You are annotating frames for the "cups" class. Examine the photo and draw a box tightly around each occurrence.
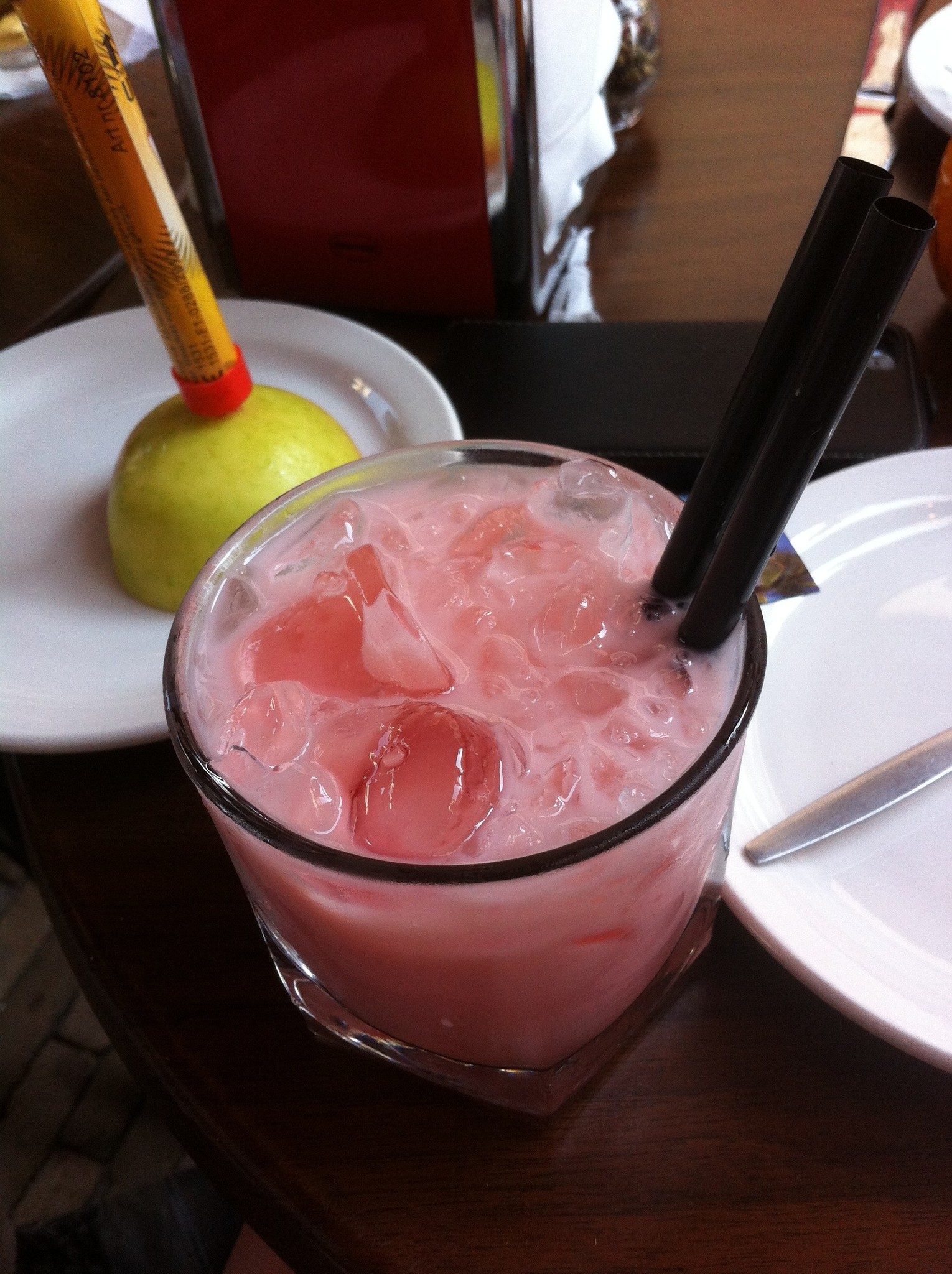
[162,441,769,1117]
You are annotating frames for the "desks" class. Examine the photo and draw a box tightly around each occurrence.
[3,309,951,1273]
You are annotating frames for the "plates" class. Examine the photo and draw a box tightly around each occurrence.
[1,300,466,755]
[722,447,952,1073]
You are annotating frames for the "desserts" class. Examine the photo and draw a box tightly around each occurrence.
[107,381,374,612]
